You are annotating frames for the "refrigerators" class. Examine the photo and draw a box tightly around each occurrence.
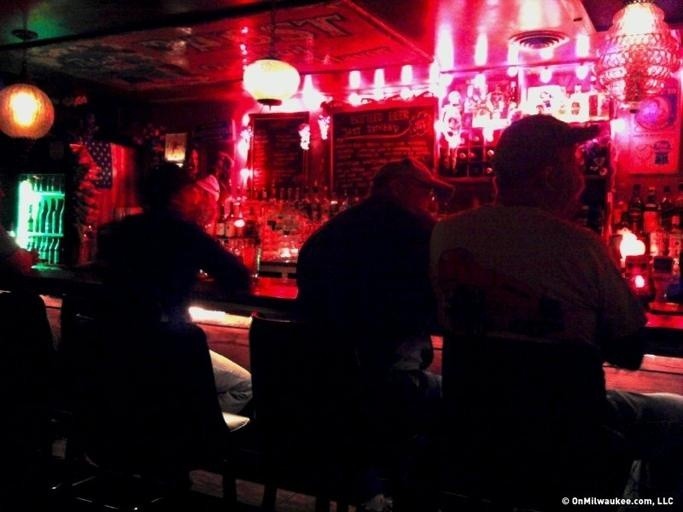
[9,135,136,269]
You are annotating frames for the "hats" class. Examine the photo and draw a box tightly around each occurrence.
[372,153,453,200]
[491,113,600,202]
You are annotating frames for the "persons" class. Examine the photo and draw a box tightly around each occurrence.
[428,111,683,512]
[292,161,455,512]
[91,160,255,493]
[0,181,40,277]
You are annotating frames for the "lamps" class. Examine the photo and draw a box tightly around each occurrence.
[240,0,300,109]
[0,26,54,141]
[596,0,681,115]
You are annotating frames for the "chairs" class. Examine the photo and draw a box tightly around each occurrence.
[216,312,437,510]
[50,277,253,511]
[439,278,649,508]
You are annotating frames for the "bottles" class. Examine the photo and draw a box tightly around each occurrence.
[615,181,683,259]
[23,173,66,264]
[217,182,356,287]
[567,76,600,121]
[441,103,462,147]
